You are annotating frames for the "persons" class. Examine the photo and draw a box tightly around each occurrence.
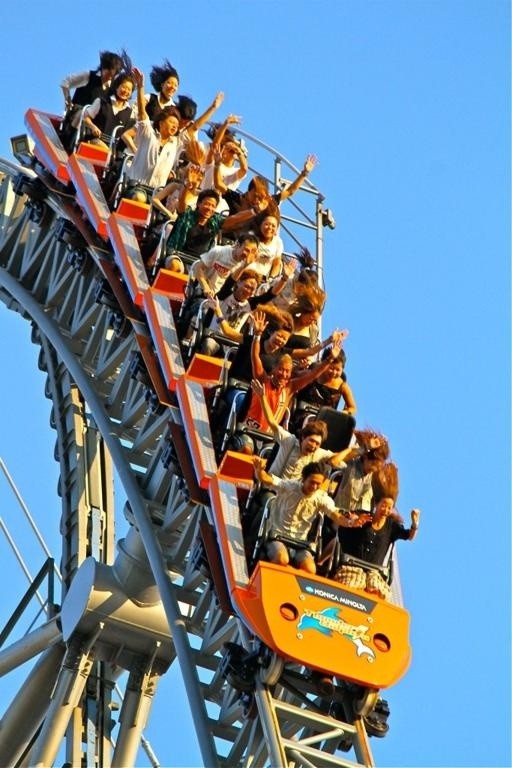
[64,48,421,594]
